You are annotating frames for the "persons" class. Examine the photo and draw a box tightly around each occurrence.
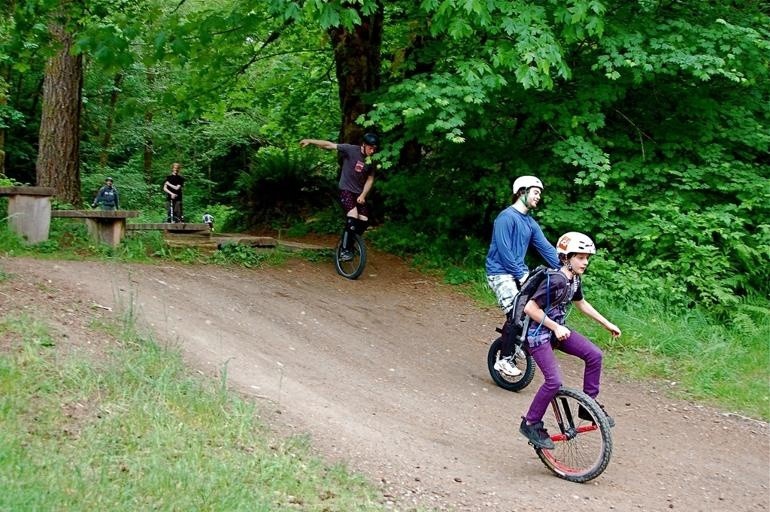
[202,212,216,231]
[162,164,186,224]
[92,177,119,211]
[518,230,622,450]
[484,175,560,376]
[299,132,380,261]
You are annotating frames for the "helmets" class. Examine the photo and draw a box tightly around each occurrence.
[362,132,379,146]
[555,231,598,255]
[511,174,545,196]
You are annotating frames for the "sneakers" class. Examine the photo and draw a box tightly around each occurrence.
[339,246,355,261]
[491,349,522,377]
[513,344,527,359]
[518,415,556,449]
[577,399,617,427]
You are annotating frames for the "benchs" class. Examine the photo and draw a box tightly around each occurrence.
[51,210,139,247]
[0,186,55,244]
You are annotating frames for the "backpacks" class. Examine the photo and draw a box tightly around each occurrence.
[508,263,581,362]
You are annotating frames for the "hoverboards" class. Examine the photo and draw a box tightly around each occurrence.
[527,342,614,483]
[487,326,536,393]
[333,203,368,280]
[163,197,185,223]
